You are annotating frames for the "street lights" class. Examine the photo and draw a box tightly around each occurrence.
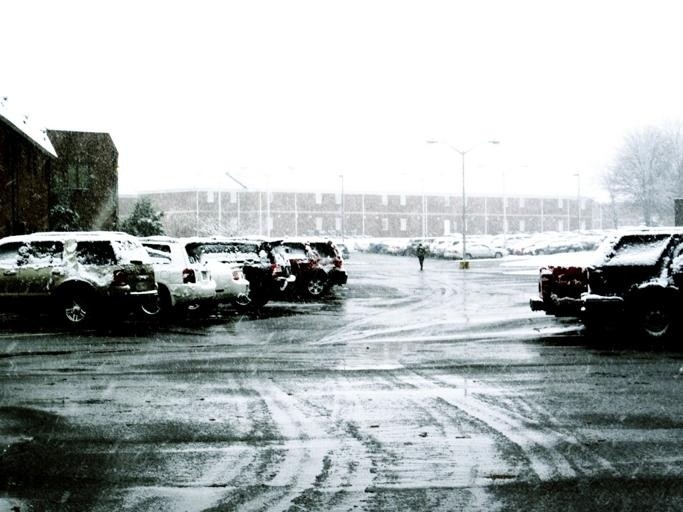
[427,137,500,259]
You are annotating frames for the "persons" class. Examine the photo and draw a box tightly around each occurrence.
[416,244,427,273]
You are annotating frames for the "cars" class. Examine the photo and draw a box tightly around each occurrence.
[355,231,595,259]
[529,225,683,343]
